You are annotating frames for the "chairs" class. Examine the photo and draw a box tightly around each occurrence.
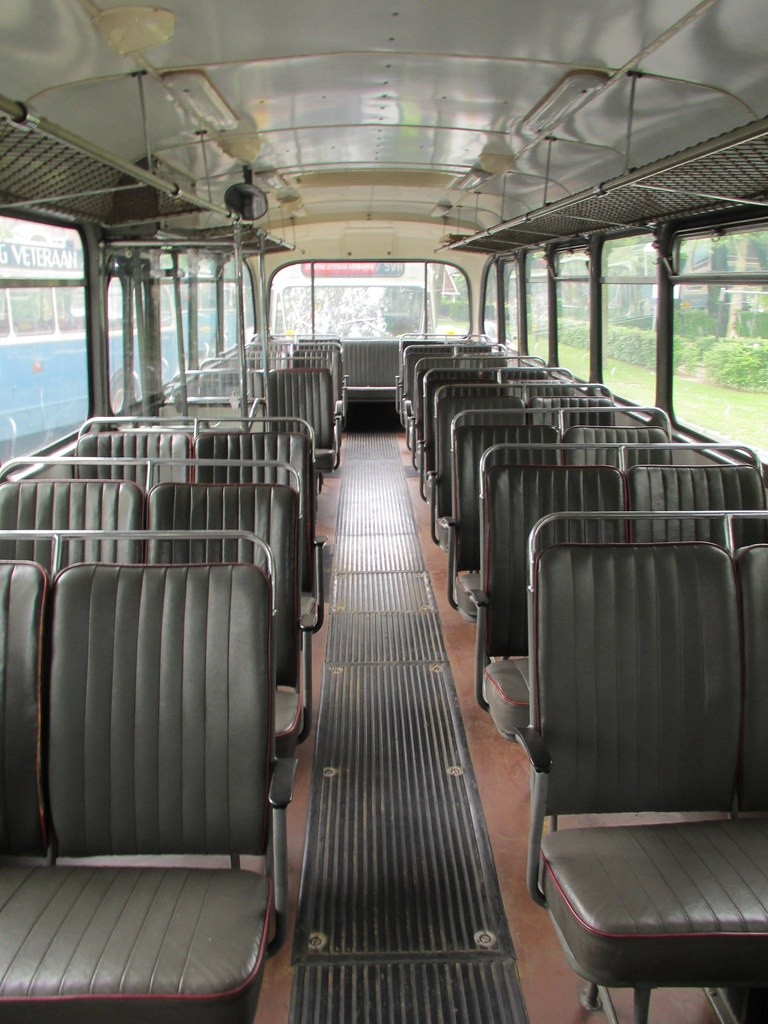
[0,341,768,1024]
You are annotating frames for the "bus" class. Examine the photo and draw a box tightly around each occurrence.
[0,241,245,450]
[267,262,437,354]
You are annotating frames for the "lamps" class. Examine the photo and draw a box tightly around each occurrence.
[522,69,610,136]
[429,204,452,218]
[452,167,491,191]
[161,69,239,132]
[261,173,291,190]
